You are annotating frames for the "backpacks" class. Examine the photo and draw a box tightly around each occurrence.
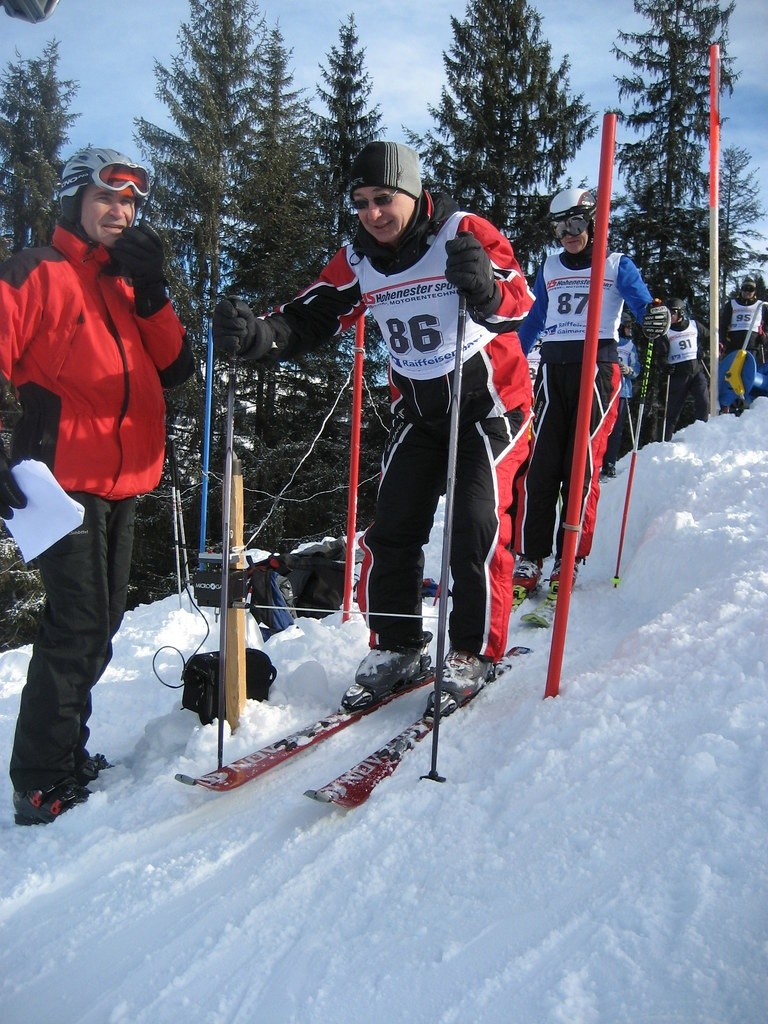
[246,536,346,642]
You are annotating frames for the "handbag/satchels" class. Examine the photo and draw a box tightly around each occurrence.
[181,647,277,726]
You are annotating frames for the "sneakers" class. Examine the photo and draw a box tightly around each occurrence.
[549,556,579,593]
[433,646,490,698]
[73,753,116,783]
[355,648,421,693]
[12,778,93,826]
[601,461,618,478]
[512,555,543,591]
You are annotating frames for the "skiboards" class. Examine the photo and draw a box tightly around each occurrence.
[171,645,533,811]
[509,582,559,627]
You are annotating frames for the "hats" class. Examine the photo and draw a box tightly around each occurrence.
[740,276,756,287]
[349,141,422,198]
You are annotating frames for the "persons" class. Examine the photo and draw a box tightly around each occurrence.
[601,279,768,478]
[0,148,195,826]
[212,141,537,700]
[517,188,671,596]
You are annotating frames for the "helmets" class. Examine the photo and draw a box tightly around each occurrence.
[618,312,631,331]
[60,148,133,206]
[549,189,596,238]
[666,297,686,310]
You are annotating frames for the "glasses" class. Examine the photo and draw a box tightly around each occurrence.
[550,216,590,239]
[625,322,638,330]
[670,309,678,316]
[91,162,150,198]
[350,189,398,210]
[741,285,756,293]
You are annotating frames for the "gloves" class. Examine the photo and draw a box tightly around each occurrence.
[0,440,28,521]
[212,296,258,362]
[755,333,767,345]
[113,219,170,315]
[443,237,501,321]
[618,363,629,375]
[641,303,671,339]
[660,362,676,375]
